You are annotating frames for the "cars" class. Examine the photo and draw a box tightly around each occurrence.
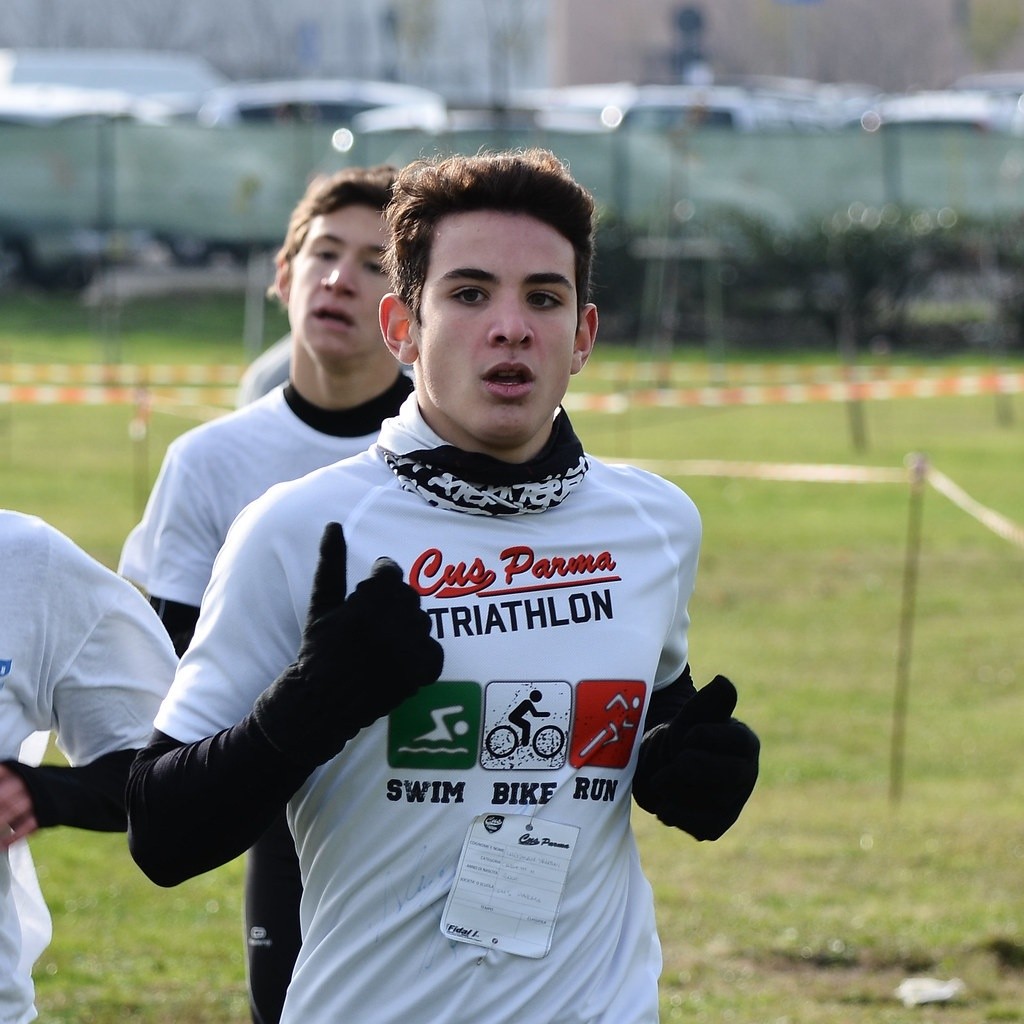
[0,47,1024,302]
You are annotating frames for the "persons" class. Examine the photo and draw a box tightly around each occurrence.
[0,509,183,1024]
[129,148,761,1024]
[117,166,415,1022]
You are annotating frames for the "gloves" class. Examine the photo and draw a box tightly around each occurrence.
[634,674,762,843]
[253,521,445,773]
